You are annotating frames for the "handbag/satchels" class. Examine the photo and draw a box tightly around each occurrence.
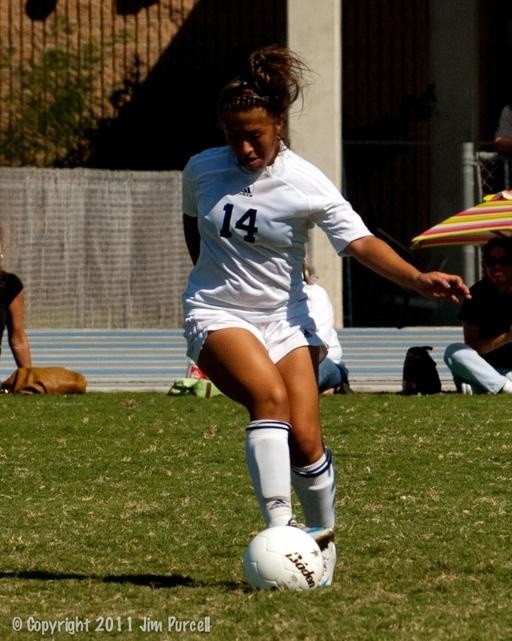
[0,365,89,397]
[165,376,225,399]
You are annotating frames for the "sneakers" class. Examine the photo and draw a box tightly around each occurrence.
[286,517,335,552]
[319,534,337,588]
[333,381,355,394]
[459,382,478,398]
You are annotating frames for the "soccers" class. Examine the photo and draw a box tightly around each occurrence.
[244,525,324,595]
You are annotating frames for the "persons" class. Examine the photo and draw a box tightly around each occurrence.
[176,43,472,593]
[0,245,33,371]
[440,234,511,397]
[301,260,353,397]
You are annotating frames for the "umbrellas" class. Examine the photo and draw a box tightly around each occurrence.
[410,188,512,249]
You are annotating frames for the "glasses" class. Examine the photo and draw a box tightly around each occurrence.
[482,254,511,270]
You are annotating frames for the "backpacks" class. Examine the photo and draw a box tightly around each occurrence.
[397,344,442,396]
